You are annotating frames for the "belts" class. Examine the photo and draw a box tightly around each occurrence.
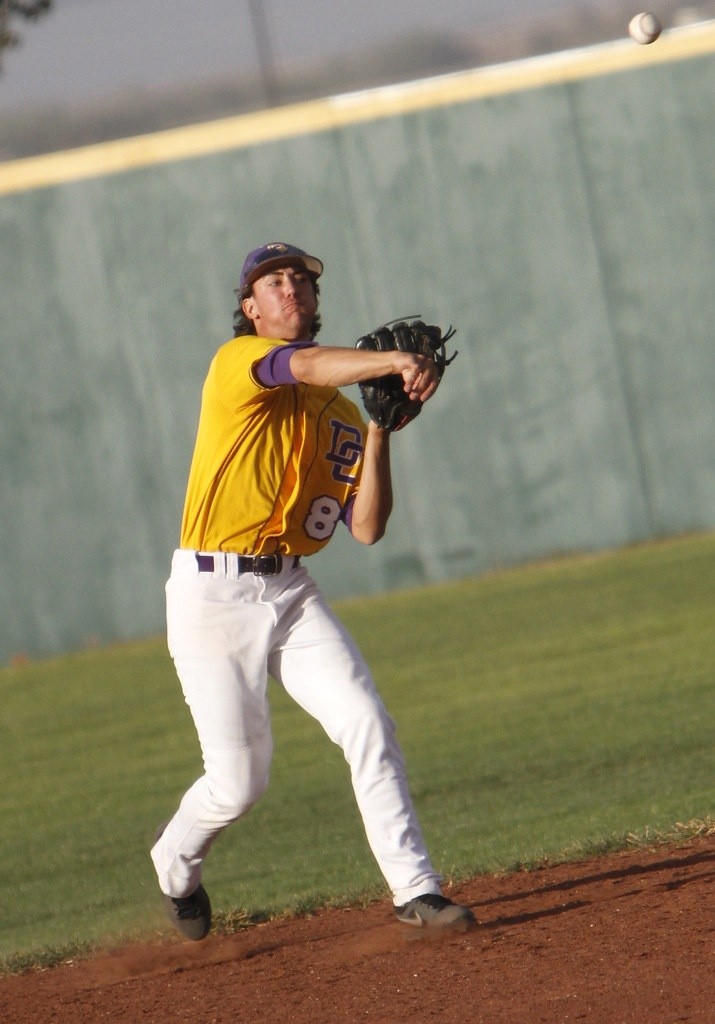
[197,554,301,576]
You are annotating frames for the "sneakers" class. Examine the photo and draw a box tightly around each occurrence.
[153,822,212,940]
[393,893,476,935]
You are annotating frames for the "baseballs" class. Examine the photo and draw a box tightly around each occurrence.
[627,11,663,45]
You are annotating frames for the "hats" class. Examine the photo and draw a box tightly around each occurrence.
[239,241,323,295]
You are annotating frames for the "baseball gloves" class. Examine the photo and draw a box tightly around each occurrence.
[354,319,445,433]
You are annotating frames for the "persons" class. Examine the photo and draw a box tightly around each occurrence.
[149,242,475,943]
